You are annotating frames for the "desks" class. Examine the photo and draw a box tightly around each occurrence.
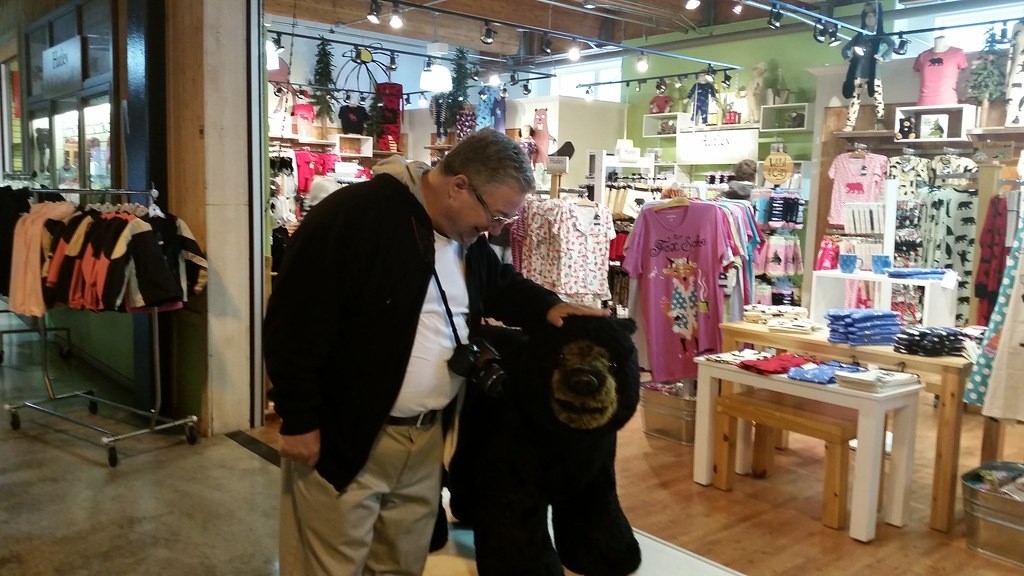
[718,307,1009,534]
[692,348,927,543]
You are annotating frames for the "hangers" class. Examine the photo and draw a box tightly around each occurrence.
[609,176,752,233]
[270,189,298,229]
[0,176,187,231]
[832,145,979,176]
[521,187,609,212]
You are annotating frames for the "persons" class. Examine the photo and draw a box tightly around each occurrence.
[913,36,968,106]
[750,63,769,123]
[842,1,895,132]
[261,133,612,576]
[682,69,720,128]
[715,160,757,200]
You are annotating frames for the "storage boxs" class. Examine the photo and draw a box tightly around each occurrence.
[638,380,696,446]
[962,461,1024,568]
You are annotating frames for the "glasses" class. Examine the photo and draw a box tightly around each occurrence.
[470,182,520,225]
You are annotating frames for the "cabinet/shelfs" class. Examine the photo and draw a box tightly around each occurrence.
[743,188,803,302]
[809,269,959,332]
[753,161,811,200]
[585,143,691,181]
[424,144,455,151]
[840,182,928,328]
[831,104,1024,143]
[269,134,404,158]
[640,102,816,139]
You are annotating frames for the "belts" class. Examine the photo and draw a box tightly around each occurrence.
[385,411,444,428]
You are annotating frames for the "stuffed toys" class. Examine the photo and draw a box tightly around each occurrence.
[426,313,652,576]
[897,116,916,140]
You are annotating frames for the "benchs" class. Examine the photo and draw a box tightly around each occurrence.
[713,389,890,531]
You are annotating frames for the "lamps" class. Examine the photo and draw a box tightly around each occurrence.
[265,1,914,104]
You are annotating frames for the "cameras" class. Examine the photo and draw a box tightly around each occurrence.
[448,337,506,394]
